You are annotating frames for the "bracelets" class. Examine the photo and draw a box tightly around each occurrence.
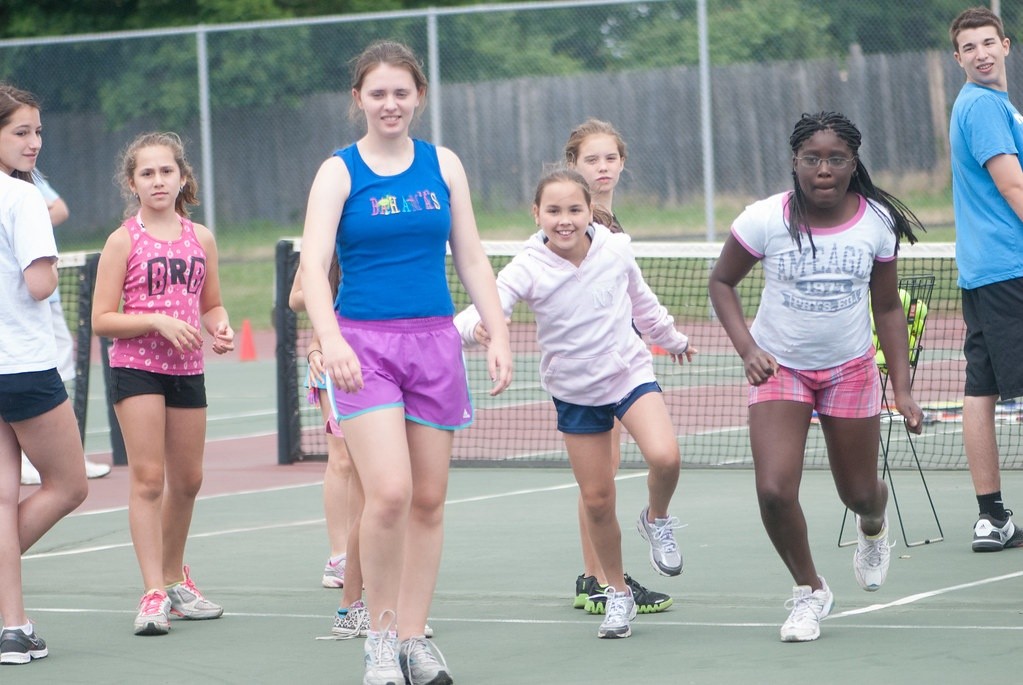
[307,350,322,364]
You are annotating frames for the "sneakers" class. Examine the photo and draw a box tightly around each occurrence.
[322,557,364,590]
[134,589,171,635]
[0,623,48,664]
[972,509,1023,552]
[573,572,673,614]
[165,565,223,619]
[637,506,688,577]
[598,585,637,638]
[333,600,433,640]
[363,609,406,685]
[399,636,453,685]
[781,575,835,642]
[854,511,896,591]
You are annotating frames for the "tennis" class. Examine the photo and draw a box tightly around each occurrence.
[867,286,927,372]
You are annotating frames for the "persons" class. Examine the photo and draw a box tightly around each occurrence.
[287,250,434,638]
[707,112,923,642]
[0,80,109,665]
[451,168,698,641]
[948,9,1022,552]
[563,118,674,613]
[299,39,515,685]
[90,132,234,635]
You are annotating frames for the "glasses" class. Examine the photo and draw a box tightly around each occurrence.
[795,155,855,169]
